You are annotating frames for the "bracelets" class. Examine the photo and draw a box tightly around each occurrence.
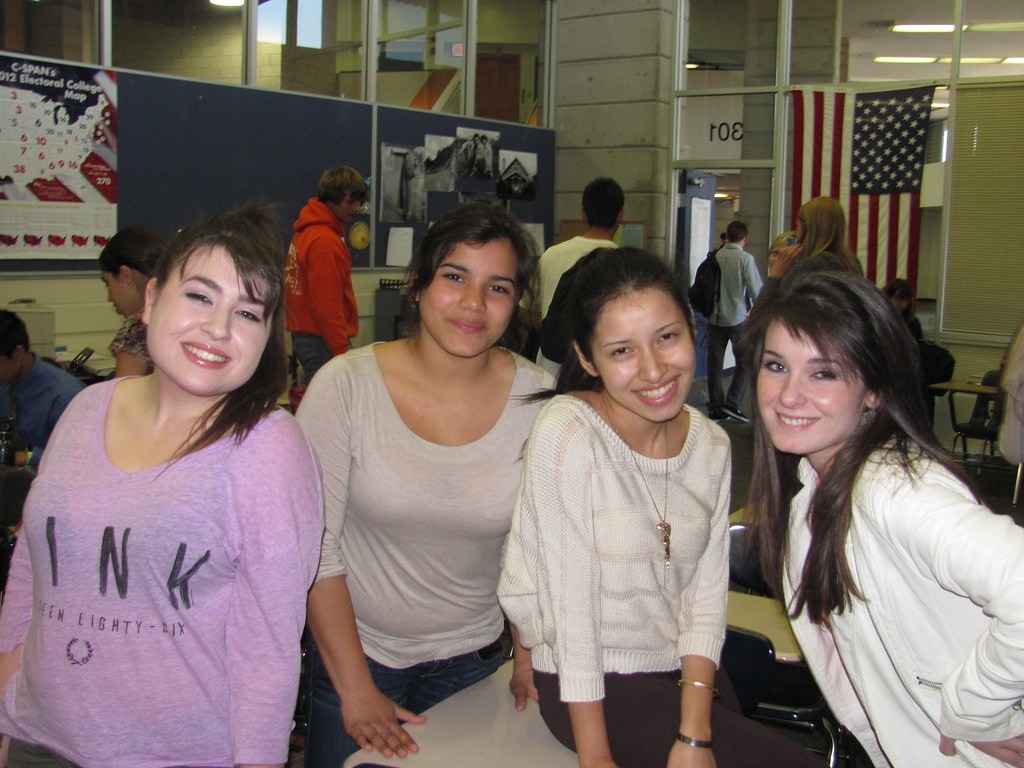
[677,732,712,749]
[678,679,718,697]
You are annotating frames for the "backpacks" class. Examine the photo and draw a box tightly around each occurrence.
[688,249,721,319]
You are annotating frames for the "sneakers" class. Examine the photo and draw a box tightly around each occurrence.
[721,404,750,422]
[708,412,731,423]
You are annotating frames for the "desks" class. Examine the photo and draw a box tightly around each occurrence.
[727,591,803,662]
[344,660,579,768]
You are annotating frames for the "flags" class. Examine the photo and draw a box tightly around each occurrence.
[791,84,934,302]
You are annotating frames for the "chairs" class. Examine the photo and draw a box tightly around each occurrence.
[950,366,1024,507]
[719,522,851,768]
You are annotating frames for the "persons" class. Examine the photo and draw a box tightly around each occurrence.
[719,232,728,245]
[703,220,762,424]
[881,278,934,428]
[996,320,1024,509]
[535,175,626,381]
[748,196,862,329]
[462,133,496,178]
[496,245,829,768]
[95,224,168,379]
[296,200,559,768]
[283,165,369,383]
[0,309,87,495]
[0,202,326,768]
[730,271,1023,767]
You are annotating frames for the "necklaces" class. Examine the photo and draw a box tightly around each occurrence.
[600,391,672,565]
